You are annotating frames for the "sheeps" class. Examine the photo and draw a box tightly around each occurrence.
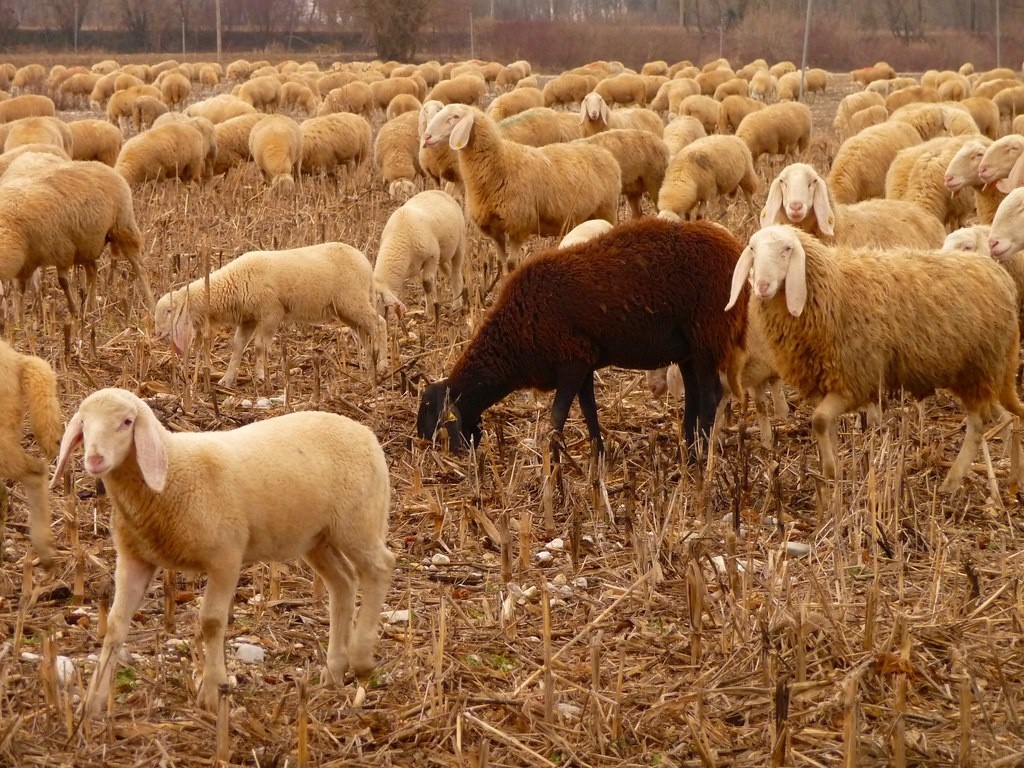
[1,53,1024,502]
[44,384,397,716]
